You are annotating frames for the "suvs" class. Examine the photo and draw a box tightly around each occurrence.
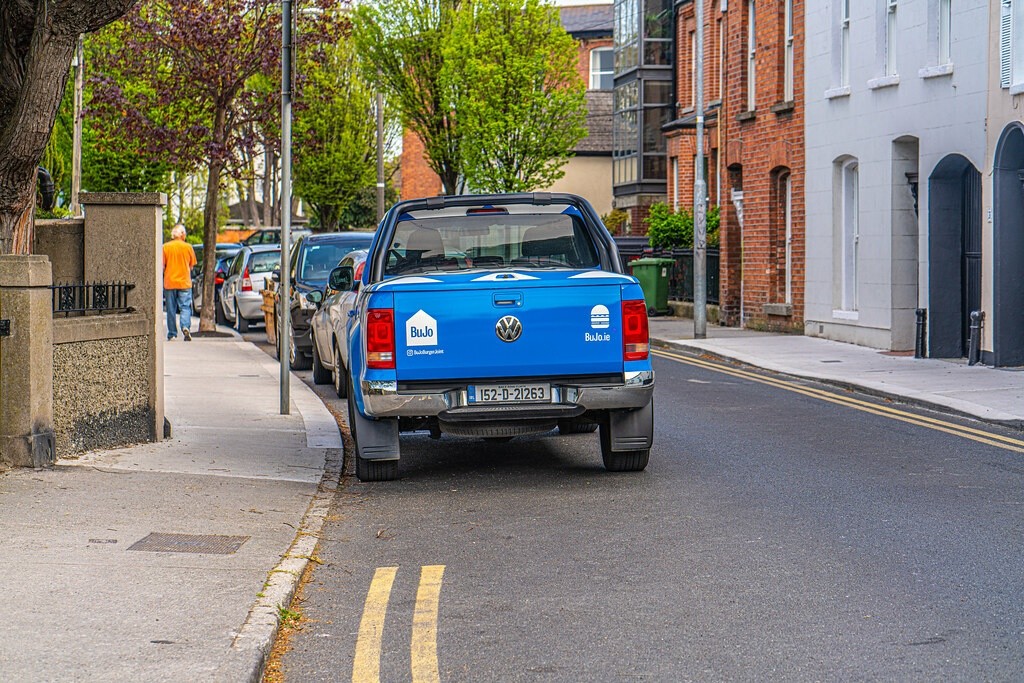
[270,231,401,371]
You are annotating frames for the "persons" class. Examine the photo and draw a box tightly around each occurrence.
[163,225,197,342]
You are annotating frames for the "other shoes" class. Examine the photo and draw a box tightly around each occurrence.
[169,336,176,340]
[182,326,191,341]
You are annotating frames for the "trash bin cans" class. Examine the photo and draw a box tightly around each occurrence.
[627,257,676,317]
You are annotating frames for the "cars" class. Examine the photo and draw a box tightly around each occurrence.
[305,246,474,399]
[161,227,312,320]
[217,242,297,333]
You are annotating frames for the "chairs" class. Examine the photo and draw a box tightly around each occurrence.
[522,225,572,267]
[398,228,458,274]
[308,249,331,278]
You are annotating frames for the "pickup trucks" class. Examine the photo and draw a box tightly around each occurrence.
[326,191,658,484]
